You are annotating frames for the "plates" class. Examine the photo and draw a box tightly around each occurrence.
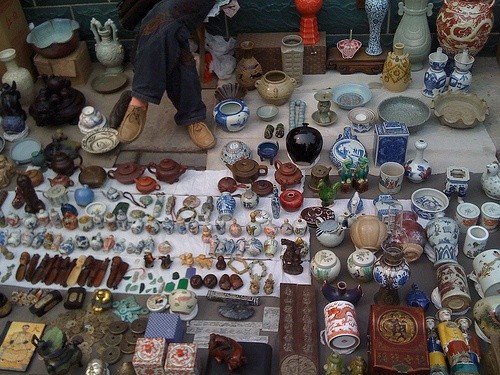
[82,128,121,154]
[10,138,41,163]
[91,72,128,94]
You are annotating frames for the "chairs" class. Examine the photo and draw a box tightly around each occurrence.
[195,0,229,89]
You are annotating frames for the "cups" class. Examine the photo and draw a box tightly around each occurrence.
[463,226,489,258]
[473,249,500,297]
[379,162,405,194]
[437,264,471,313]
[455,203,480,233]
[324,301,360,353]
[480,202,500,232]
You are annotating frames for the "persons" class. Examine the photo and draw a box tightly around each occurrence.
[117,0,217,150]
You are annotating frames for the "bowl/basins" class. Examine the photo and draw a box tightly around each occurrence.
[331,84,372,110]
[377,96,431,130]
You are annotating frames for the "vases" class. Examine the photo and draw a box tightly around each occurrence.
[349,215,389,251]
[373,242,412,305]
[397,211,426,262]
[0,48,36,115]
[426,212,459,268]
[216,192,235,221]
[236,41,262,91]
[212,98,249,132]
[364,0,496,93]
[294,0,324,45]
[404,139,431,183]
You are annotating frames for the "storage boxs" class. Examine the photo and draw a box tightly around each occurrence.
[143,312,185,343]
[0,0,29,72]
[132,337,167,375]
[164,343,201,375]
[234,31,326,74]
[33,40,91,85]
[366,304,431,375]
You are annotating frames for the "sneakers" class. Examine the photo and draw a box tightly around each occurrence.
[185,122,215,149]
[117,103,148,144]
